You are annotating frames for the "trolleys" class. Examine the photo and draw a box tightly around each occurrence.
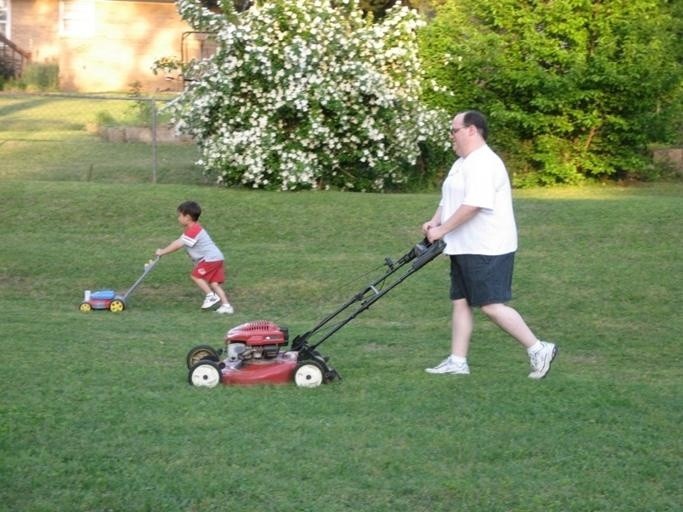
[76,250,165,313]
[185,230,447,395]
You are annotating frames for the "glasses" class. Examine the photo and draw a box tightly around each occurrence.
[450,125,469,137]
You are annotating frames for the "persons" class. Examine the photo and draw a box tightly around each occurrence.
[154,202,236,314]
[421,110,556,380]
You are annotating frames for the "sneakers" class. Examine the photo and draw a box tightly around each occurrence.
[527,341,558,379]
[425,356,470,375]
[214,304,234,315]
[200,291,221,309]
